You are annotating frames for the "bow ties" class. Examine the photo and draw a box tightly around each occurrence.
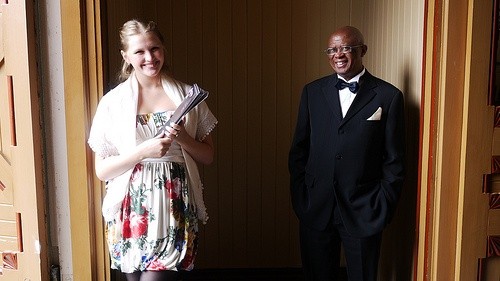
[336,78,357,94]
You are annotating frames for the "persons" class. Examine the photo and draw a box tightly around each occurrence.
[287,27,405,281]
[88,18,222,280]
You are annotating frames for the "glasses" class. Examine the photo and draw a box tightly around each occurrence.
[323,44,363,55]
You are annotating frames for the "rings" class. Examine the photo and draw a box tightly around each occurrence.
[175,132,179,137]
